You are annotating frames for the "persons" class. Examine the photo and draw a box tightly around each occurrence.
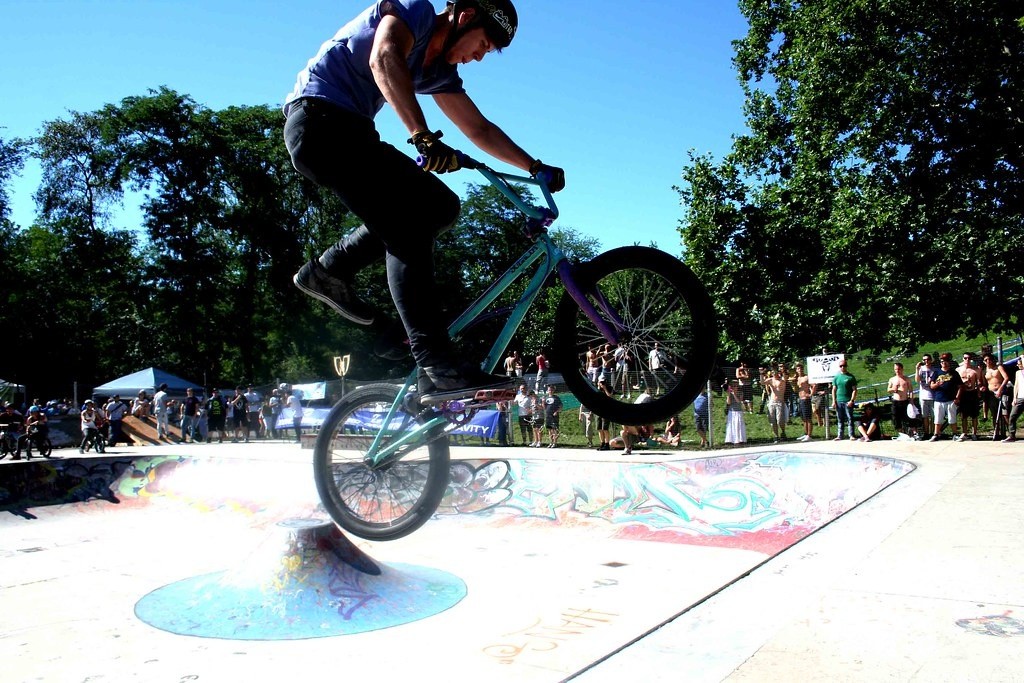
[0,398,81,460]
[736,361,753,414]
[479,405,491,446]
[831,360,857,441]
[282,0,565,405]
[79,383,304,454]
[725,380,747,444]
[887,343,1024,443]
[586,343,678,400]
[693,387,714,447]
[579,381,681,455]
[504,351,522,377]
[495,401,508,447]
[857,402,882,442]
[535,351,548,395]
[452,434,465,445]
[759,361,832,444]
[514,384,563,448]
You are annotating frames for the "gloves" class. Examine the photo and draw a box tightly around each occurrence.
[413,131,463,174]
[529,159,565,193]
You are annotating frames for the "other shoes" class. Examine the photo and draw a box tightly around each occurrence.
[295,440,301,444]
[621,447,632,455]
[11,454,21,460]
[159,434,251,444]
[519,435,1015,448]
[79,447,83,454]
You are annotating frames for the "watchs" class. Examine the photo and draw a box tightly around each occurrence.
[850,400,854,402]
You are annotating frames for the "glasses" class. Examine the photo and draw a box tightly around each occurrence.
[983,358,991,362]
[923,359,930,362]
[840,365,846,368]
[963,357,972,360]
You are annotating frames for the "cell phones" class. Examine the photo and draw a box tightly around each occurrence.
[920,363,926,365]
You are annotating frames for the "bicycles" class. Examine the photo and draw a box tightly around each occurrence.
[82,419,108,453]
[313,150,719,541]
[0,421,53,461]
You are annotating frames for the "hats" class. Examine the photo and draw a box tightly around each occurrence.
[212,387,218,393]
[247,384,253,387]
[940,354,949,360]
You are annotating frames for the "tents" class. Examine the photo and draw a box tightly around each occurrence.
[93,367,203,400]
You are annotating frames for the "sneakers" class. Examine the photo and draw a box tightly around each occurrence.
[293,257,376,325]
[418,361,515,405]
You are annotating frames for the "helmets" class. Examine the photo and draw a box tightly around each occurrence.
[447,0,518,48]
[30,406,39,412]
[85,399,92,405]
